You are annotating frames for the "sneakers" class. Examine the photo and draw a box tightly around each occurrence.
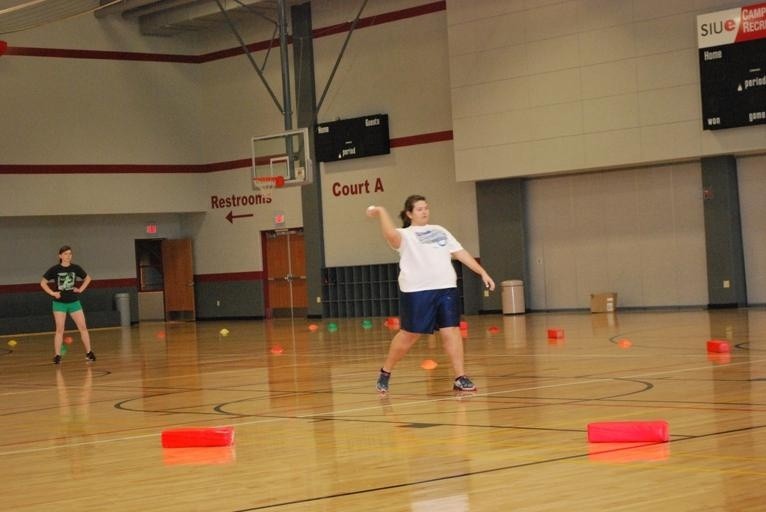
[377,368,389,392]
[86,352,96,361]
[453,375,476,390]
[53,355,61,363]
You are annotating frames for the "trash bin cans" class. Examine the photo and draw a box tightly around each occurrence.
[115,293,132,326]
[501,279,525,314]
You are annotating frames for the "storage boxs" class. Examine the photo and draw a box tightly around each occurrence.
[589,290,618,314]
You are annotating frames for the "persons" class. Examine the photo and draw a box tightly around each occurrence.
[46,361,100,478]
[366,193,496,395]
[377,389,480,511]
[40,244,97,364]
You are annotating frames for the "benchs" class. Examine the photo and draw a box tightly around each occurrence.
[1,288,121,335]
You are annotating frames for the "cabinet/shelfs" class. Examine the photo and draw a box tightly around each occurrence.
[320,260,482,317]
